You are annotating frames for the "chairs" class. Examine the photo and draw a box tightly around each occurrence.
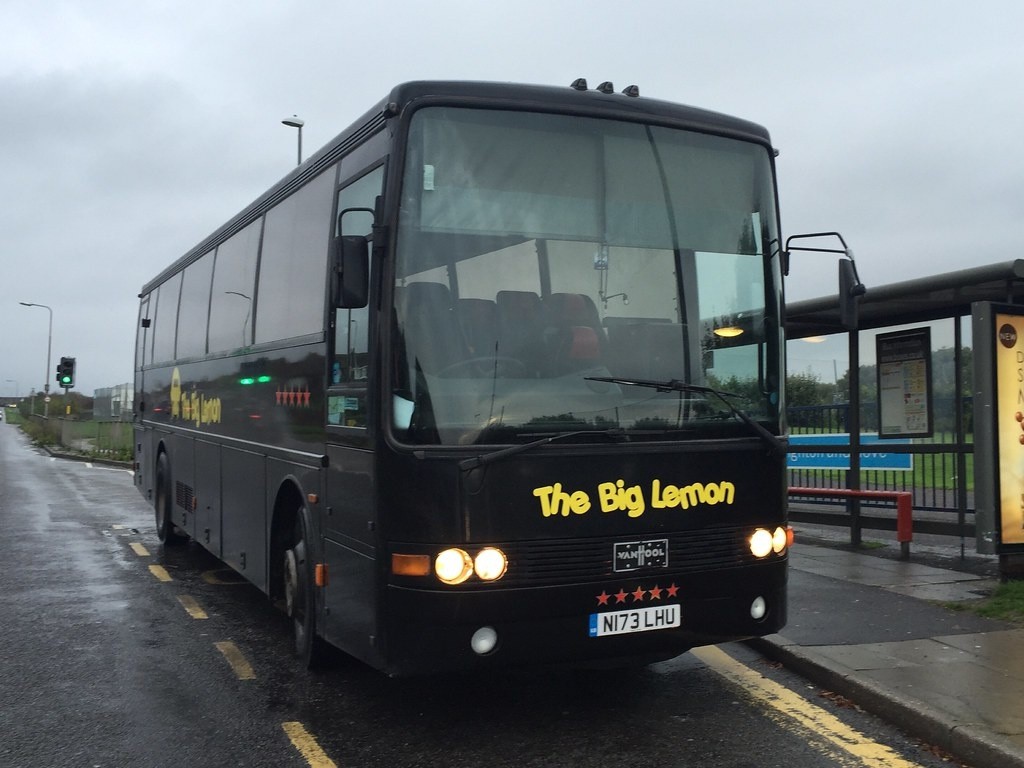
[396,282,609,382]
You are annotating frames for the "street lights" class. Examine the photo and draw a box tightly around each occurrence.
[279,114,304,168]
[18,302,53,417]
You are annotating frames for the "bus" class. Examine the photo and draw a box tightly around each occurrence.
[132,79,866,681]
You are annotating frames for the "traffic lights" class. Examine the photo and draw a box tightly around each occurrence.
[56,358,60,385]
[61,358,75,387]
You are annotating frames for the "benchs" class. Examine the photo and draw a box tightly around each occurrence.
[789,487,913,558]
